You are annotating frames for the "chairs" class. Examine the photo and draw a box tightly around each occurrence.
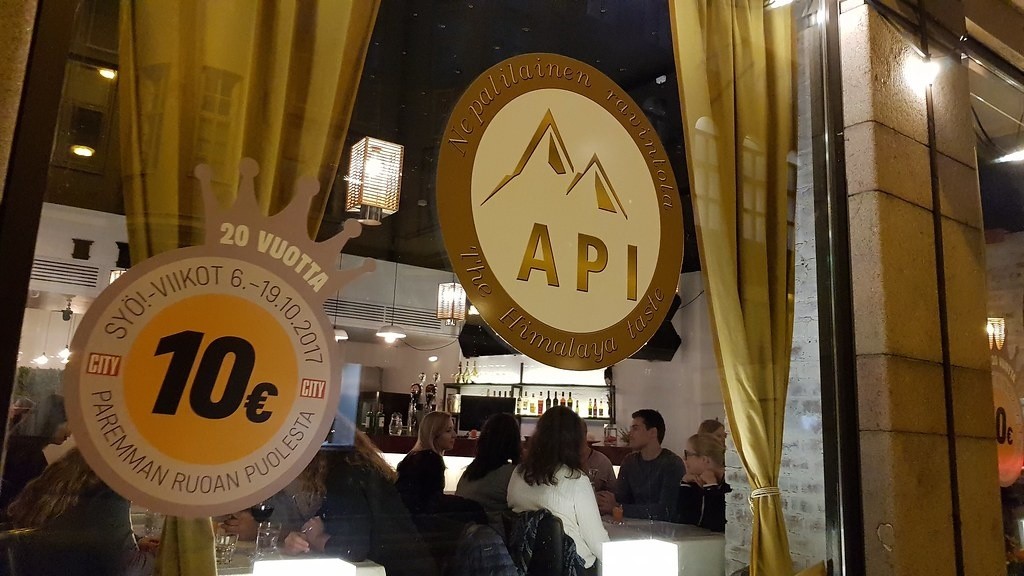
[0,522,119,576]
[411,489,588,575]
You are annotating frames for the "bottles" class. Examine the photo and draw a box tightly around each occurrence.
[599,401,603,417]
[517,391,536,414]
[560,392,579,415]
[389,414,402,436]
[328,430,335,443]
[588,399,597,418]
[486,389,512,398]
[454,361,479,383]
[538,390,558,416]
[366,404,385,436]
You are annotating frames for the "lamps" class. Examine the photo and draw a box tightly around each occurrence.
[36,310,77,365]
[375,239,406,344]
[347,81,404,226]
[109,268,127,285]
[437,271,466,326]
[332,251,348,343]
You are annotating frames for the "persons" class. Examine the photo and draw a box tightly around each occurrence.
[595,409,732,534]
[454,412,524,504]
[0,393,437,576]
[507,406,617,576]
[396,411,457,496]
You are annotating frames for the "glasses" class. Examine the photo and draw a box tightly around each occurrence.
[685,450,710,460]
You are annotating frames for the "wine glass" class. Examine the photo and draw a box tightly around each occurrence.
[247,496,275,557]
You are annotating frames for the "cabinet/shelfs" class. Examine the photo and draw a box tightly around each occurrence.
[443,383,616,424]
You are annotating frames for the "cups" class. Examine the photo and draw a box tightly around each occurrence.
[215,534,239,565]
[257,522,282,555]
[613,504,623,521]
[144,510,166,538]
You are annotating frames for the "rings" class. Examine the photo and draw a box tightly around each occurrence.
[302,528,307,533]
[309,526,312,531]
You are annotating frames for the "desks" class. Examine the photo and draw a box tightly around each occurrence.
[602,514,727,576]
[133,522,385,576]
